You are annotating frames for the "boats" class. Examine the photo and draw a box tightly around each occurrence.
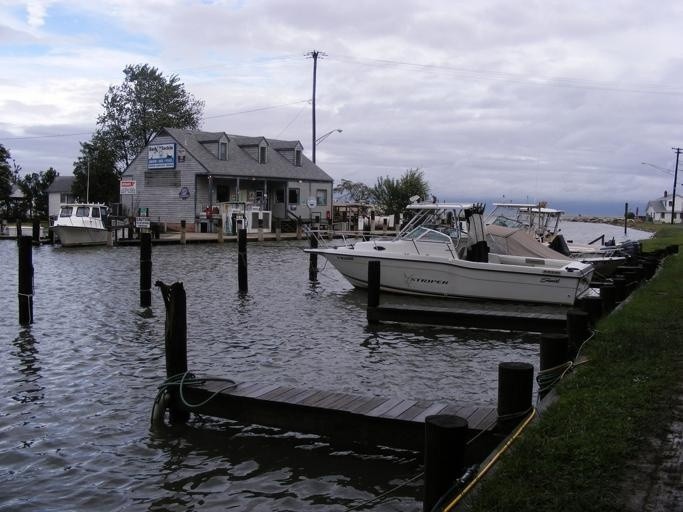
[49,201,109,246]
[303,201,602,305]
[483,203,631,289]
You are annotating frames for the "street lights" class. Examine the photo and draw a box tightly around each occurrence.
[642,161,680,223]
[312,128,343,166]
